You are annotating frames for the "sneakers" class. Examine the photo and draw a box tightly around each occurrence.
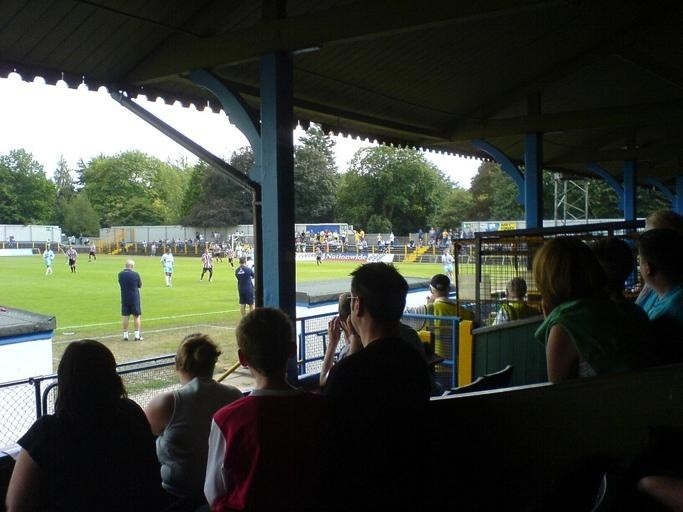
[122,331,129,341]
[134,331,144,341]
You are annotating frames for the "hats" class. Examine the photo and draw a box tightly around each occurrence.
[430,273,451,291]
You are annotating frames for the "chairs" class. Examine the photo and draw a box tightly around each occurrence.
[305,243,477,254]
[1,242,59,253]
[104,242,207,255]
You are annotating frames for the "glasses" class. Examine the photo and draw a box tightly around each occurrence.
[346,295,357,305]
